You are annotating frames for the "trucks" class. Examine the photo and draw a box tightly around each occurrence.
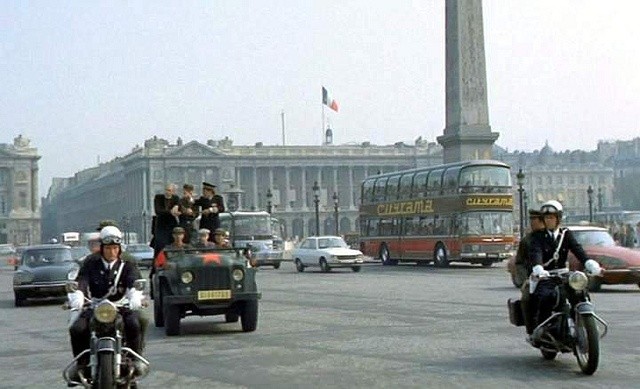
[60,232,81,247]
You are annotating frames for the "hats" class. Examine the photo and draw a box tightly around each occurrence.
[528,209,542,219]
[172,227,185,233]
[203,182,216,190]
[95,219,120,231]
[212,228,226,235]
[198,228,210,234]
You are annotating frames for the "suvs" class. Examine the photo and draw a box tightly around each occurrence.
[150,210,260,335]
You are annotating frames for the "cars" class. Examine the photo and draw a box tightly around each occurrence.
[292,236,363,273]
[562,225,640,292]
[12,244,80,308]
[125,244,158,267]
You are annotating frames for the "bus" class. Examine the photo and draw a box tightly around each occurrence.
[265,218,284,269]
[218,212,271,268]
[358,159,515,268]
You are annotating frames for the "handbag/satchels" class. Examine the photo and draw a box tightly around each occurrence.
[508,298,525,326]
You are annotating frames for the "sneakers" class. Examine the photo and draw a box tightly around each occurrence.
[68,366,91,387]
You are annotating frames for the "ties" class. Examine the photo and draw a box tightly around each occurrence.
[551,231,555,240]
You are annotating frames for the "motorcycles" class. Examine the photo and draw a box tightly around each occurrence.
[507,262,607,377]
[64,278,149,389]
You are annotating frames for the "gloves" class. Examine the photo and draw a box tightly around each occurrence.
[584,259,605,277]
[532,264,550,279]
[67,290,85,309]
[129,291,144,311]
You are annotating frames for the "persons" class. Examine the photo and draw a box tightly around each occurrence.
[362,178,509,236]
[212,227,233,249]
[516,208,546,280]
[66,219,149,389]
[527,200,603,343]
[155,228,192,268]
[154,183,180,251]
[191,229,215,249]
[609,220,640,247]
[195,182,225,233]
[178,184,195,241]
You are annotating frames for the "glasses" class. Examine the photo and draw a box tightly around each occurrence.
[541,205,557,213]
[101,236,122,245]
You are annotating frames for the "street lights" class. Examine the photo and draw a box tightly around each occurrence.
[311,181,322,236]
[265,188,274,214]
[517,169,525,239]
[331,192,340,235]
[587,186,594,223]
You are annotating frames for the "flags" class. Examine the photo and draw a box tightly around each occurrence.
[322,87,338,112]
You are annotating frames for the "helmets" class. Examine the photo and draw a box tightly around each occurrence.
[540,200,563,219]
[99,226,123,253]
